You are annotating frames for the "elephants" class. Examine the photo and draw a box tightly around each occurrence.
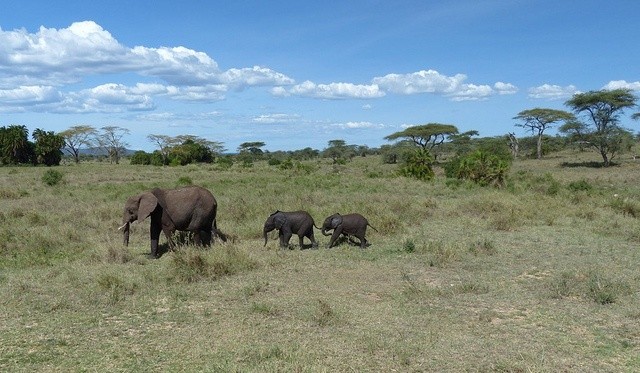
[322,212,379,250]
[117,185,227,261]
[262,209,322,252]
